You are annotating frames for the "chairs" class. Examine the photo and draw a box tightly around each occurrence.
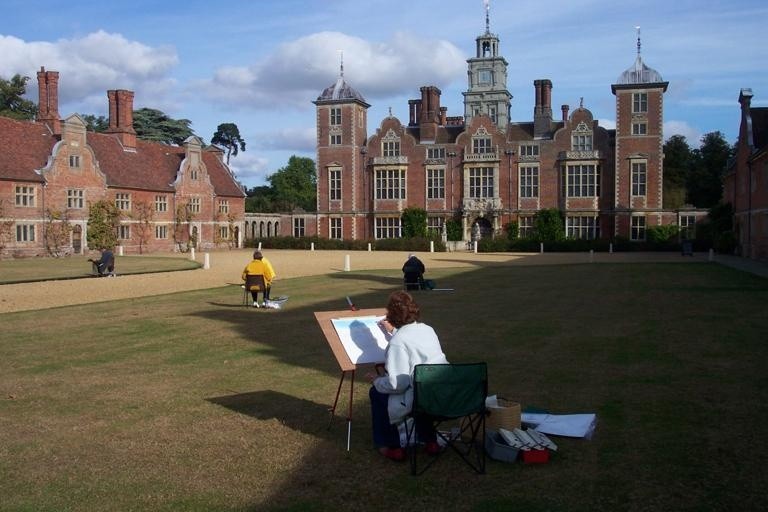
[403,264,425,293]
[404,362,490,474]
[242,275,267,309]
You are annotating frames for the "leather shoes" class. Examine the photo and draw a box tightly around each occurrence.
[379,446,405,461]
[262,302,269,307]
[254,302,259,308]
[424,442,440,456]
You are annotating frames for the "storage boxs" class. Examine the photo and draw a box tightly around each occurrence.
[485,431,519,462]
[524,449,549,464]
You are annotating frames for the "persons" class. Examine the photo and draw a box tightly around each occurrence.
[370,290,450,461]
[242,252,275,309]
[403,253,425,289]
